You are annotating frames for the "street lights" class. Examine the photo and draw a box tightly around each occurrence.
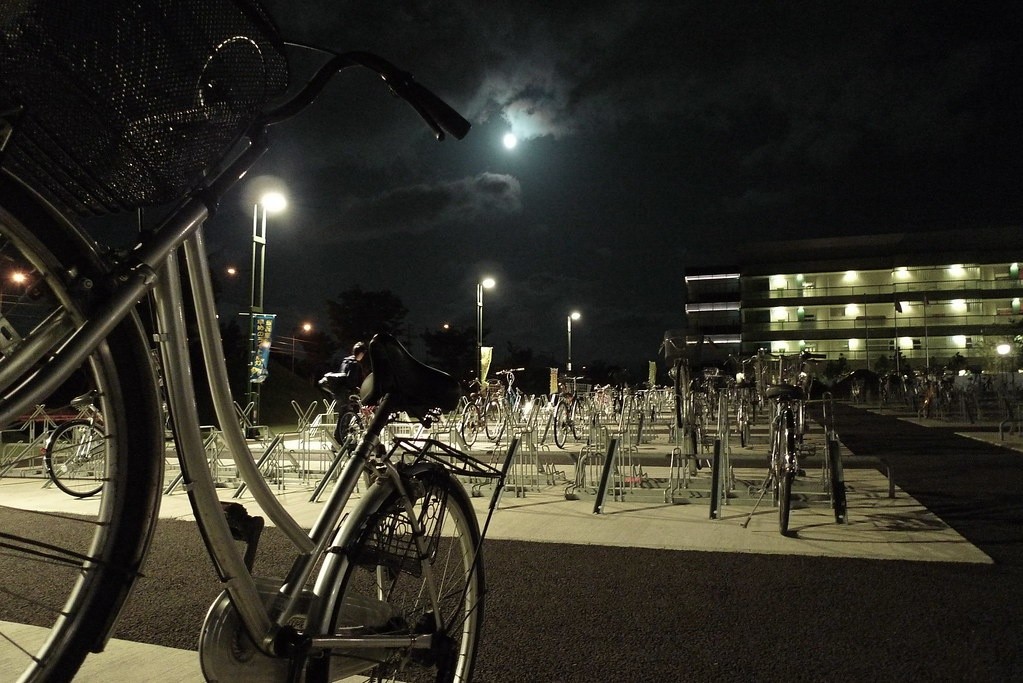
[246,191,287,425]
[475,277,496,379]
[567,312,581,377]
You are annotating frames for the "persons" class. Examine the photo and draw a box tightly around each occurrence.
[328,341,367,451]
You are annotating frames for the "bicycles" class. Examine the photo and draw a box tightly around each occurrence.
[45,347,183,497]
[0,26,514,683]
[302,346,1023,536]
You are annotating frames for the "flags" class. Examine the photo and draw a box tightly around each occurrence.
[896,296,902,313]
